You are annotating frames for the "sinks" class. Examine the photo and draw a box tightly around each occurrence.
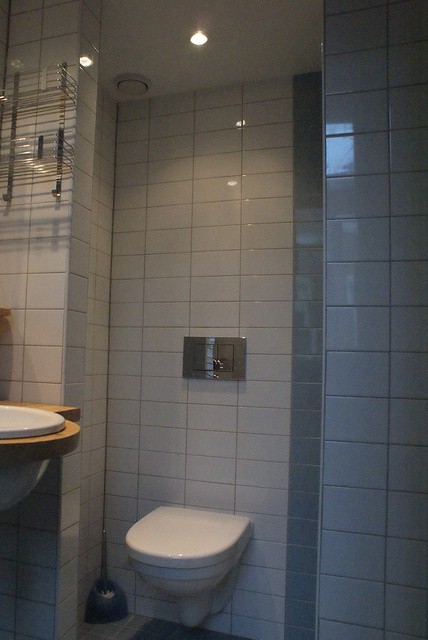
[0,400,81,512]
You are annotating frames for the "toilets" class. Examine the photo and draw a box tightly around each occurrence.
[124,505,254,628]
